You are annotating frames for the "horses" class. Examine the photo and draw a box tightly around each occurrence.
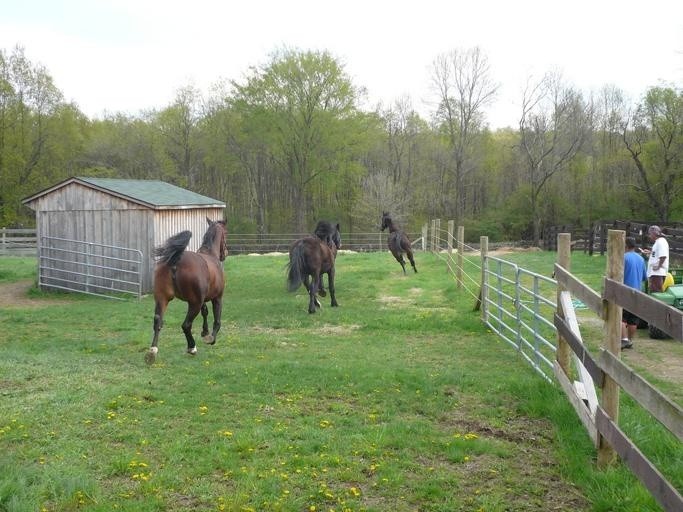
[281,220,343,312]
[144,213,229,367]
[380,210,419,276]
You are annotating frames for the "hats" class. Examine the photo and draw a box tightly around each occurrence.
[649,226,666,237]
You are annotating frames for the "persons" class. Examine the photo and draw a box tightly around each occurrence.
[647,225,669,295]
[621,238,647,348]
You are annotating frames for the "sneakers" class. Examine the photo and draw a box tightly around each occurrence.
[621,339,632,349]
